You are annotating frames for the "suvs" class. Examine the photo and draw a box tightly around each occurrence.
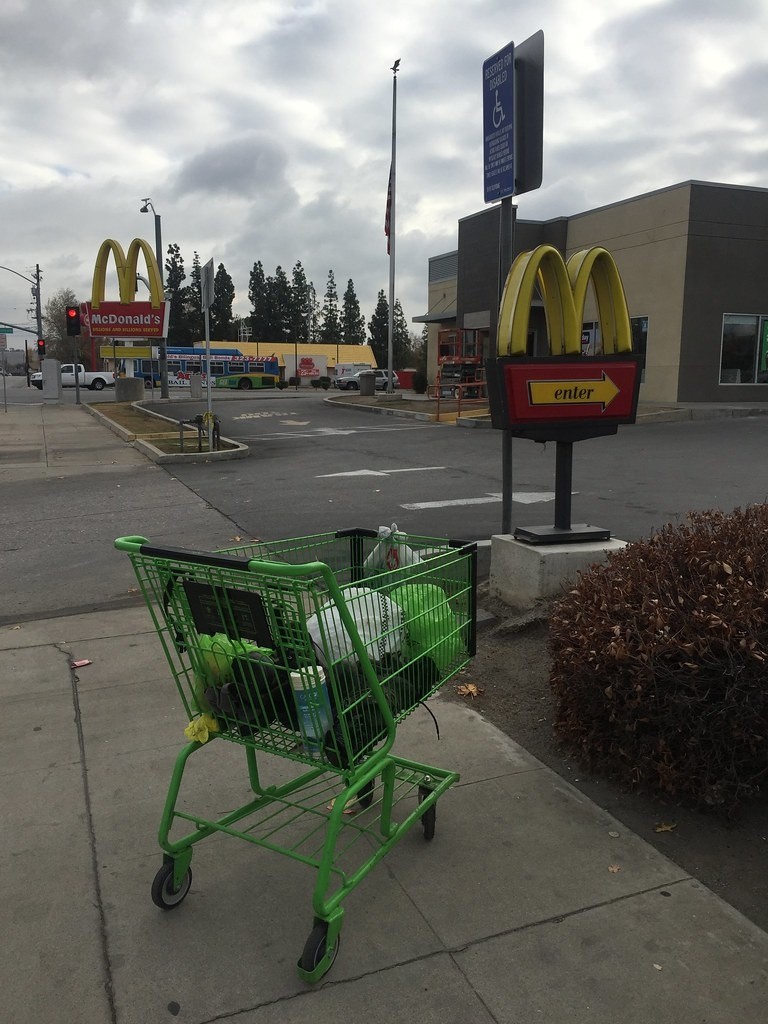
[336,368,400,391]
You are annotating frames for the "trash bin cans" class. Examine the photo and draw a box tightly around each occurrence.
[190,375,202,398]
[359,374,377,396]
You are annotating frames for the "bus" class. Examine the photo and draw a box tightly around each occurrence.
[112,346,281,392]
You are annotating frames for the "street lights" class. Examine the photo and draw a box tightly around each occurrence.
[139,196,163,286]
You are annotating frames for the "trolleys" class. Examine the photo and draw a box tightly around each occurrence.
[114,524,479,986]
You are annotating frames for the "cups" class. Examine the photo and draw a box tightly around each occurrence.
[292,666,333,759]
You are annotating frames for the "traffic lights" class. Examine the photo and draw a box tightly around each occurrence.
[65,305,81,336]
[37,339,45,355]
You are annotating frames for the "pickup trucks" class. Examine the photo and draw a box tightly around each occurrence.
[30,364,116,390]
